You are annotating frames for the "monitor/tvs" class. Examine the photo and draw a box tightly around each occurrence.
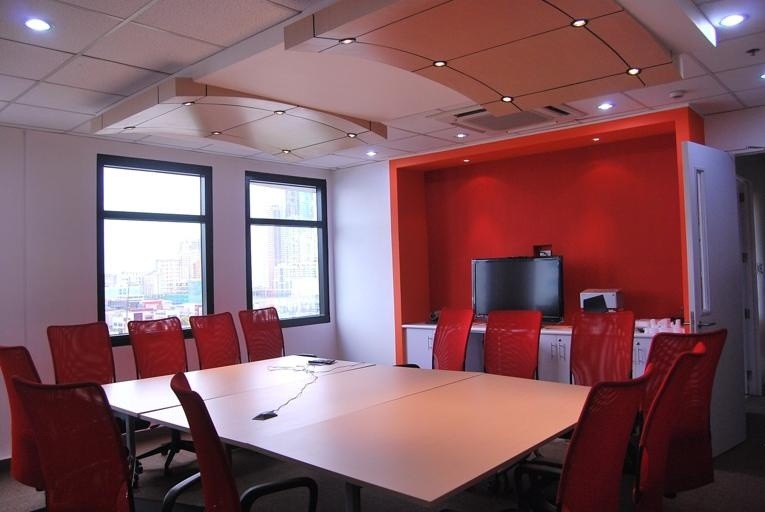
[471,256,564,325]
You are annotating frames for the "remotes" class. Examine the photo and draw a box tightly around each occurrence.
[308,359,335,365]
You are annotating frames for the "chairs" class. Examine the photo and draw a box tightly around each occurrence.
[635,339,709,512]
[238,307,285,362]
[432,308,476,370]
[127,316,196,479]
[645,330,727,398]
[169,371,319,512]
[483,309,544,380]
[46,321,143,490]
[9,375,205,511]
[0,345,46,494]
[569,311,637,383]
[558,362,659,510]
[189,312,241,370]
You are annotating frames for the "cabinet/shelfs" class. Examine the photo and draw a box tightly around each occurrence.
[402,321,485,372]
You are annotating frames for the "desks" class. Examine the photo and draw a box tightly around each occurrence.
[102,352,484,450]
[250,373,601,512]
